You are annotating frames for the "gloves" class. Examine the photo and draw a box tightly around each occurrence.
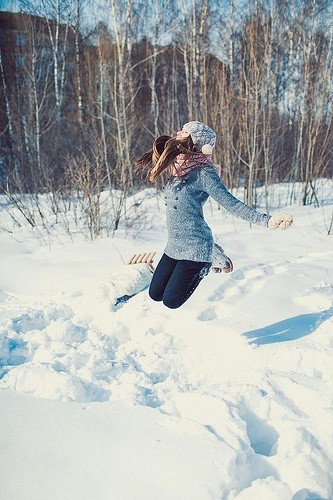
[268,213,293,230]
[212,164,221,177]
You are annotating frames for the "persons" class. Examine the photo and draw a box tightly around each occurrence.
[136,120,294,308]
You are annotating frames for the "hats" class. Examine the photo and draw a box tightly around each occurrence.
[183,121,217,153]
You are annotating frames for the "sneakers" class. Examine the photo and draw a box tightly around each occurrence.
[211,243,233,273]
